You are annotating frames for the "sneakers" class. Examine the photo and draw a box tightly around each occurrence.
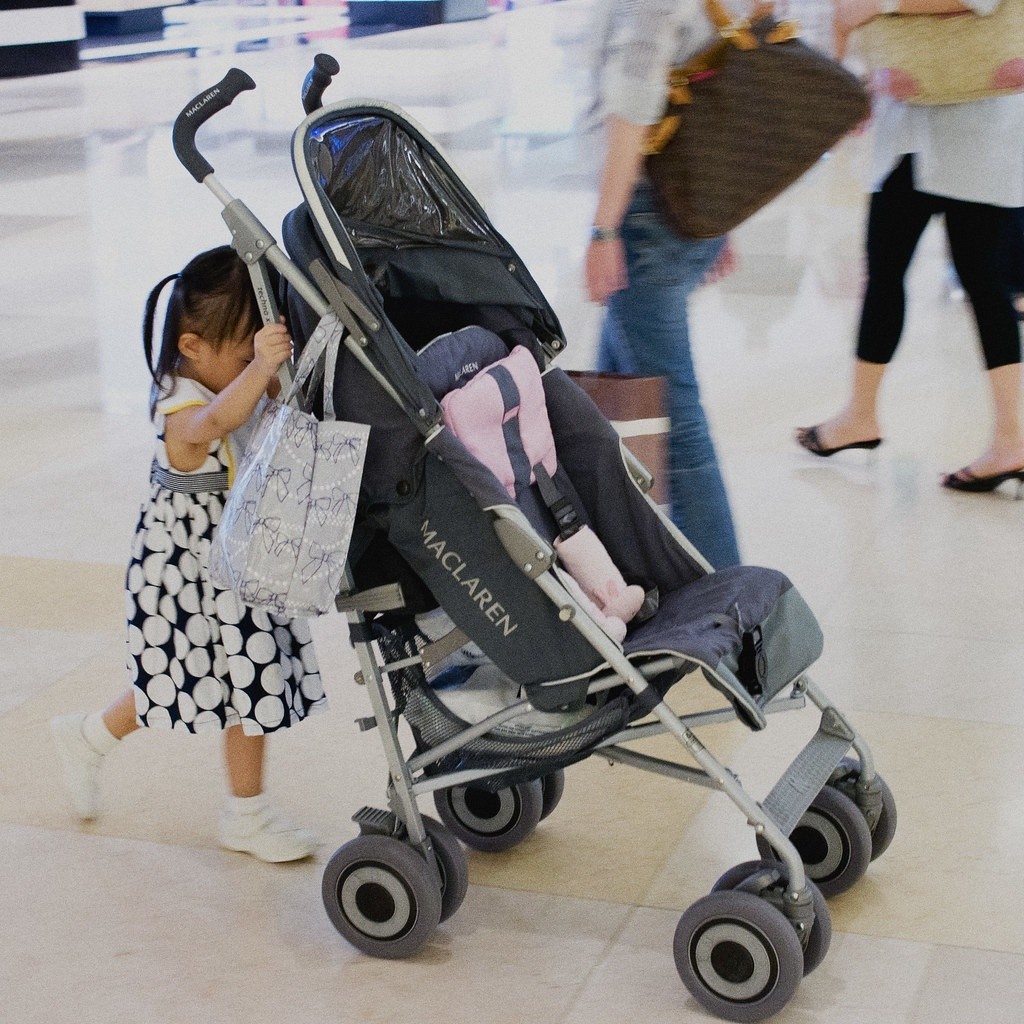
[217,805,318,866]
[55,712,106,824]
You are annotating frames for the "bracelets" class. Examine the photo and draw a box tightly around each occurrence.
[881,0,897,15]
[585,224,624,241]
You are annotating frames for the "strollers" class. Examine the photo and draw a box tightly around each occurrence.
[175,53,895,1023]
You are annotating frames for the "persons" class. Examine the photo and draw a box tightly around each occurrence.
[583,1,791,583]
[795,0,1023,501]
[57,241,329,866]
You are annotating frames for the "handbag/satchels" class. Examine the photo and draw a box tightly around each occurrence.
[862,0,1024,106]
[634,11,875,240]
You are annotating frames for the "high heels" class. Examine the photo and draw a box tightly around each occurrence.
[935,461,1024,500]
[794,417,883,466]
